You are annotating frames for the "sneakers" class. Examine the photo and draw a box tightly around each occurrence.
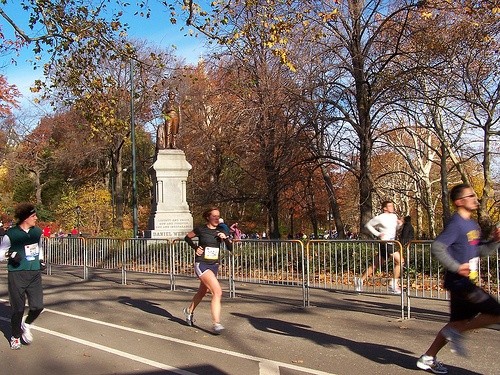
[20,315,33,344]
[416,354,448,374]
[354,277,364,294]
[385,278,402,293]
[10,336,21,349]
[441,325,469,358]
[213,324,224,334]
[183,307,194,326]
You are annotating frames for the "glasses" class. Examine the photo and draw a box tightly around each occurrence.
[455,194,476,200]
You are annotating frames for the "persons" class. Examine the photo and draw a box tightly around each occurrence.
[217,218,357,243]
[401,216,414,244]
[162,91,182,149]
[354,201,411,296]
[185,205,233,335]
[416,183,500,374]
[136,230,143,238]
[0,219,78,249]
[0,204,48,349]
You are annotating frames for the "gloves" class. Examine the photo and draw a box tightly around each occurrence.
[40,261,47,271]
[5,250,21,260]
